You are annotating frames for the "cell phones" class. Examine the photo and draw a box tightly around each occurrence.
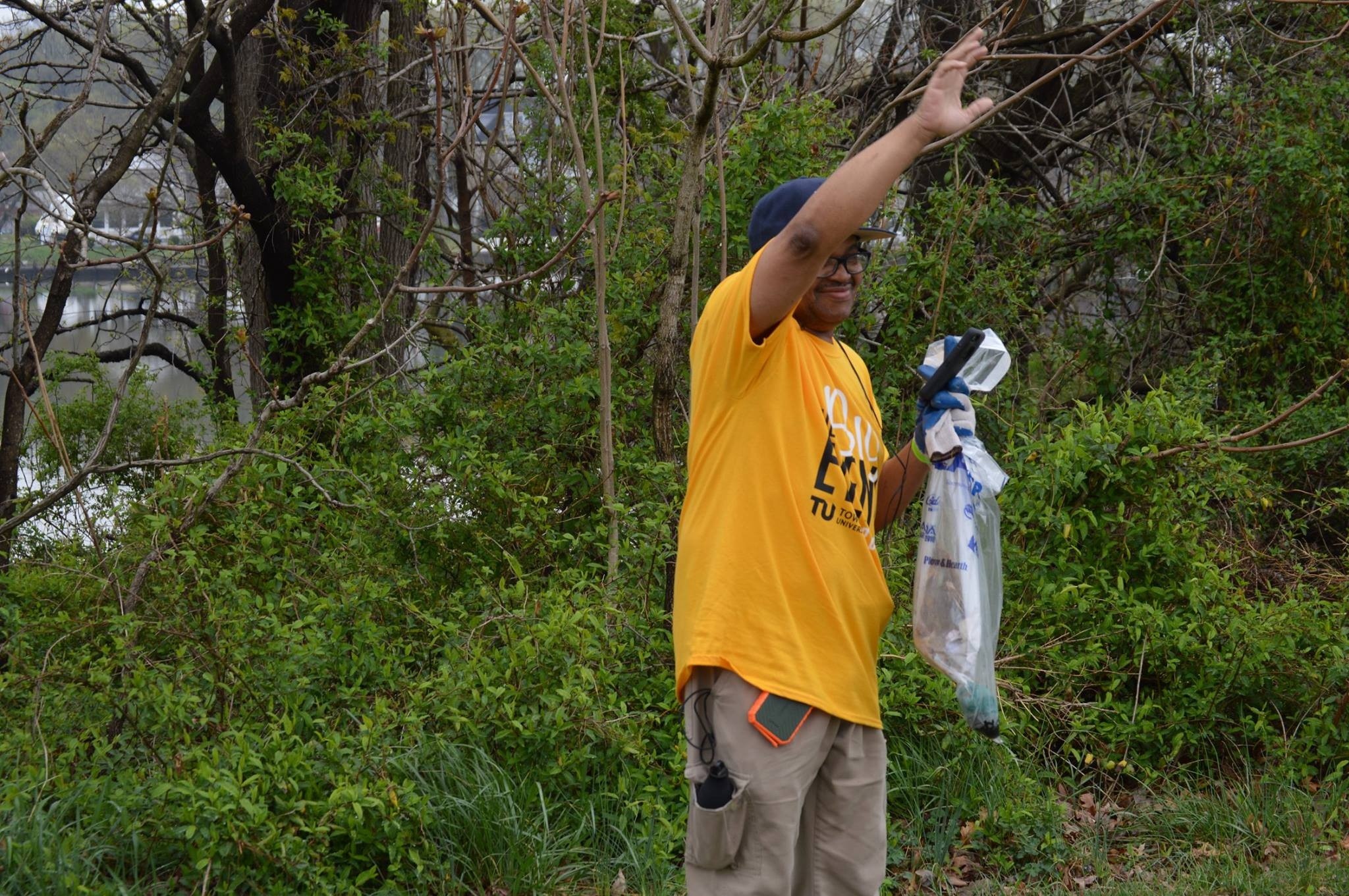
[748,691,815,747]
[919,328,986,399]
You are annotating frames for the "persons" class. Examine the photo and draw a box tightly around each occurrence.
[673,27,995,896]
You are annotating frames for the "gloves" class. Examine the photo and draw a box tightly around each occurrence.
[911,335,976,464]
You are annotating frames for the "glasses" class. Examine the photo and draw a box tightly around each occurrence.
[816,247,872,279]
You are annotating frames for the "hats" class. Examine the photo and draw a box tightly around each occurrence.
[748,176,898,256]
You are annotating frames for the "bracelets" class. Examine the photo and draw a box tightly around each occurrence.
[912,440,931,464]
